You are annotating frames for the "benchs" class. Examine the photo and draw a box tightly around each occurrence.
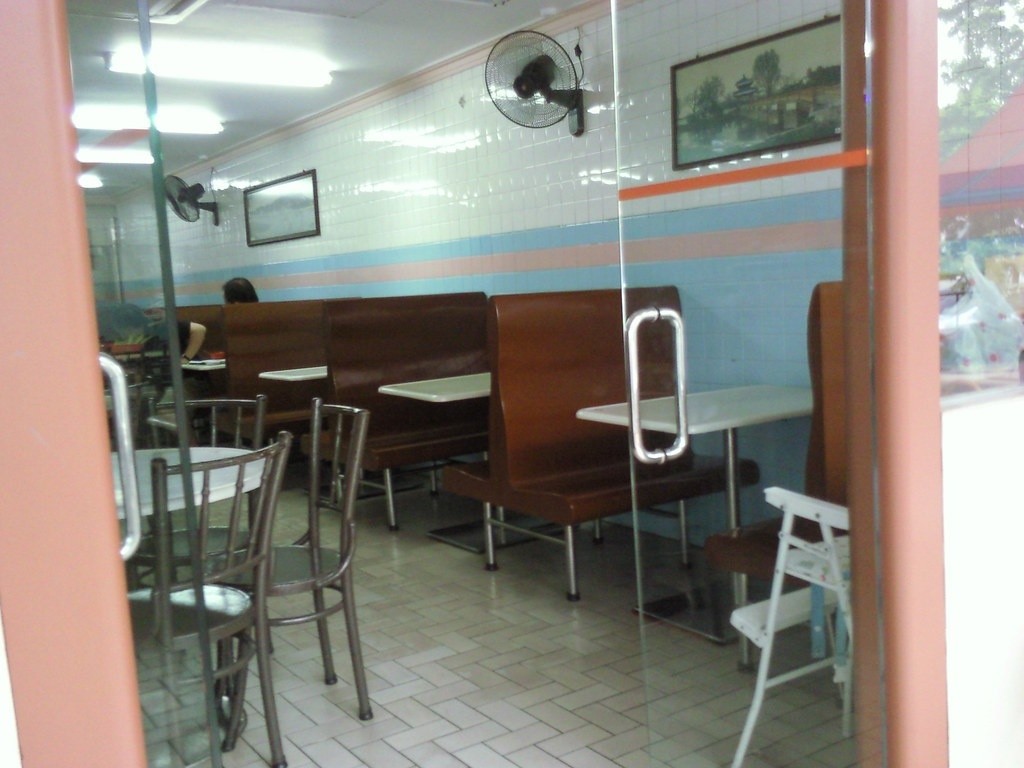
[708,274,976,670]
[139,286,759,609]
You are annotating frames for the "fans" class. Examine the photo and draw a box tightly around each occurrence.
[485,30,587,136]
[165,175,220,227]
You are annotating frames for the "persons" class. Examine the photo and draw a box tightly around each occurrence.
[221,278,259,305]
[110,303,211,447]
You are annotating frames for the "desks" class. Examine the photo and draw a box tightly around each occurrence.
[180,356,225,371]
[576,384,816,647]
[112,449,267,595]
[257,365,434,503]
[379,372,567,554]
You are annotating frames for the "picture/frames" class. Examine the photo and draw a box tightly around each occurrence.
[670,16,843,171]
[243,167,321,247]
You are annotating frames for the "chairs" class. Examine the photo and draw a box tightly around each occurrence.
[207,397,375,751]
[128,431,294,768]
[134,393,267,691]
